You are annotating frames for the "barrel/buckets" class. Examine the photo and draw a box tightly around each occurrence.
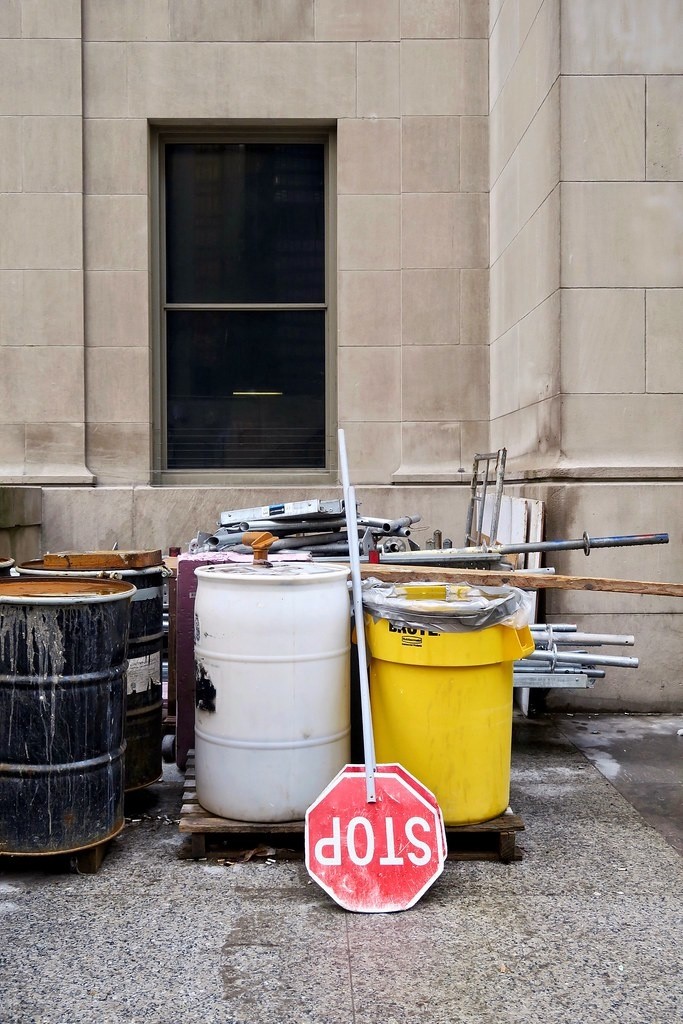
[16,559,165,791]
[0,576,125,856]
[0,557,15,576]
[192,562,350,823]
[360,582,535,827]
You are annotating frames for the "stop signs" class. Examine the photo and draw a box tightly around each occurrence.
[303,769,445,913]
